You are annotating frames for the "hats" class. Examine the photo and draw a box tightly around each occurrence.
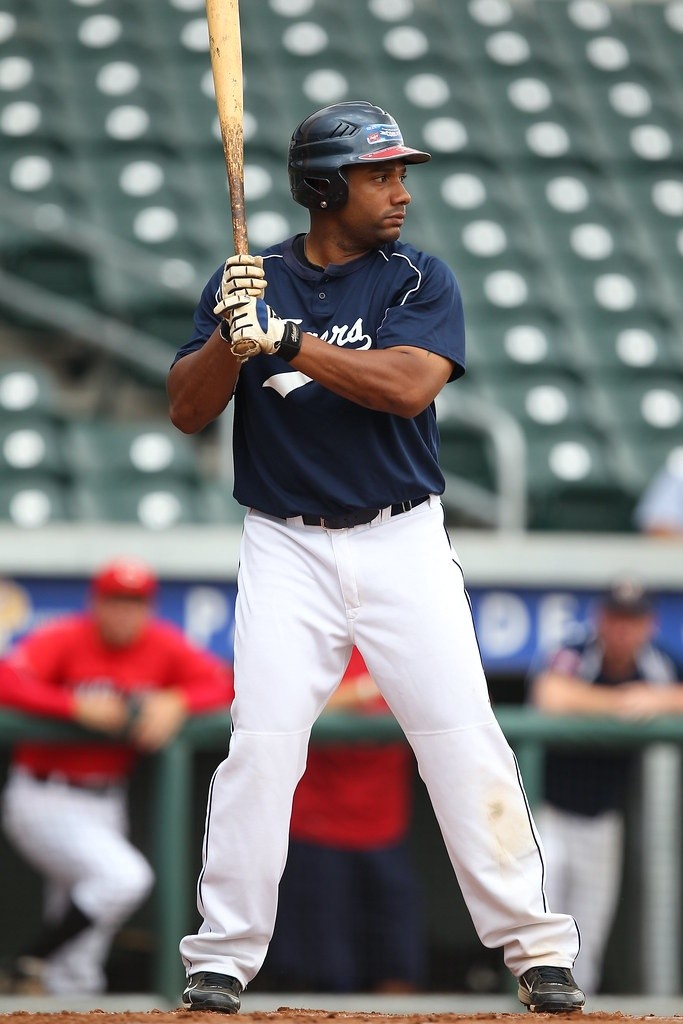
[90,560,163,607]
[598,578,655,616]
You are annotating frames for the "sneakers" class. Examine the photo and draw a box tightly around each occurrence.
[178,966,246,1014]
[514,964,589,1015]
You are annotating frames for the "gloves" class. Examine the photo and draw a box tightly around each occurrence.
[212,251,268,345]
[212,293,303,364]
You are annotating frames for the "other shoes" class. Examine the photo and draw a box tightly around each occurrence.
[12,952,61,999]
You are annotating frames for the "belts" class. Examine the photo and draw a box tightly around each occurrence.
[248,491,434,531]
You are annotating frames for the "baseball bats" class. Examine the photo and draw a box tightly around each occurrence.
[205,0,261,355]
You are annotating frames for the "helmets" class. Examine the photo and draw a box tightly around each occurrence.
[286,97,433,177]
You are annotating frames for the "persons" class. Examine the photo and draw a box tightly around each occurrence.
[0,555,235,997]
[531,574,683,998]
[288,637,426,993]
[169,97,586,1018]
